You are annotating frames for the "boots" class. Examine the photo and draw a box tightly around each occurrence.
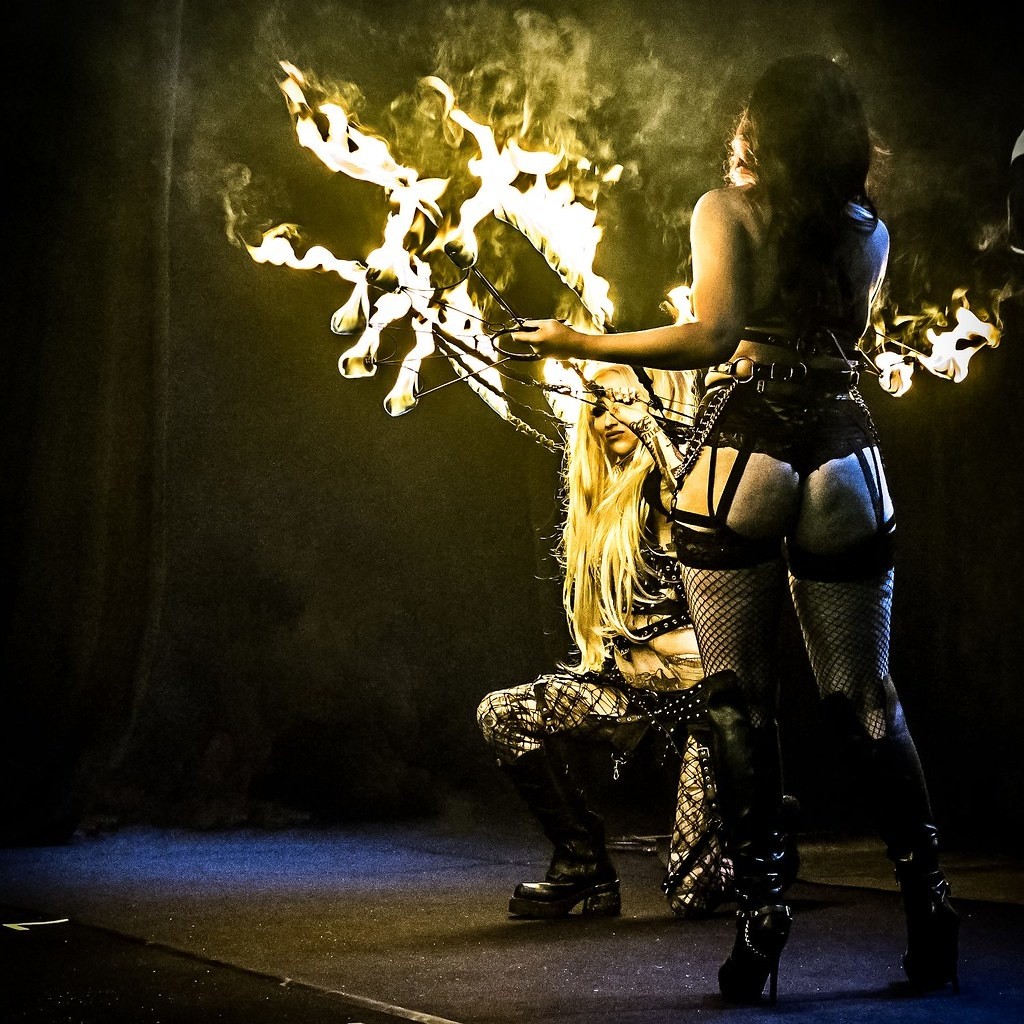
[507,746,622,917]
[781,796,800,879]
[694,670,791,1002]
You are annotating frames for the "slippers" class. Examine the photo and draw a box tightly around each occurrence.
[815,674,965,991]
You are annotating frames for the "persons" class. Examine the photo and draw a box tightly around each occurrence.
[472,354,757,920]
[509,50,977,1006]
